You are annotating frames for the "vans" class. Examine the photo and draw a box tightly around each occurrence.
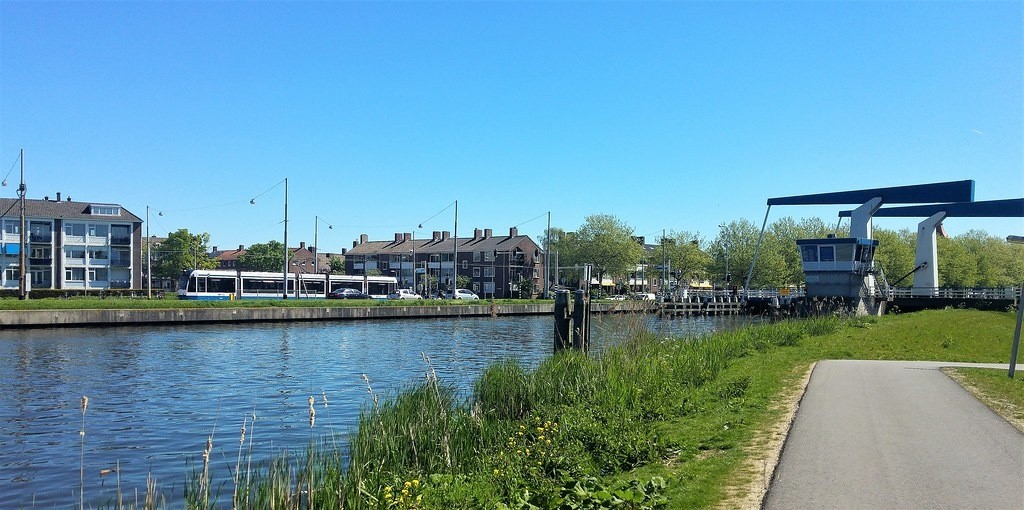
[445,288,479,300]
[591,293,656,301]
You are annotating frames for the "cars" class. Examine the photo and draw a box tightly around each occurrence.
[387,288,424,299]
[326,288,373,300]
[423,295,443,300]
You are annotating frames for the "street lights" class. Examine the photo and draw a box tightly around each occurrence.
[2,148,25,300]
[147,206,164,299]
[250,177,288,300]
[1005,235,1024,379]
[418,200,458,298]
[513,211,551,299]
[314,215,333,274]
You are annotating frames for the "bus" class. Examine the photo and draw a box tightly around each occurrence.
[177,268,400,300]
